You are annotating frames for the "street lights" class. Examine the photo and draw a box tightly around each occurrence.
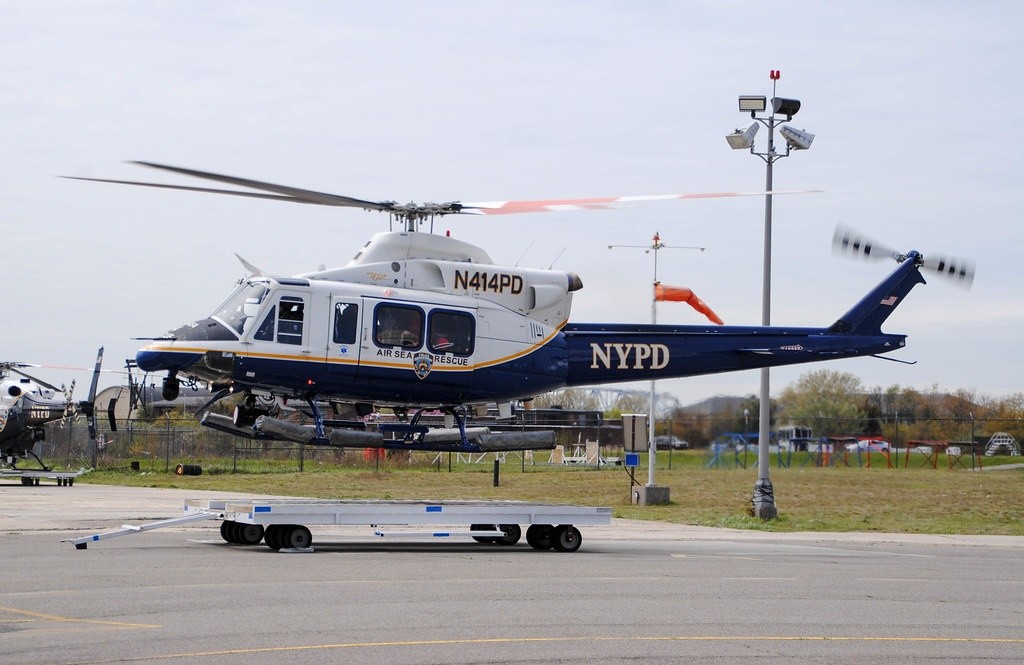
[725,70,816,522]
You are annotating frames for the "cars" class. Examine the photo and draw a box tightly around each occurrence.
[648,434,690,451]
[844,439,892,454]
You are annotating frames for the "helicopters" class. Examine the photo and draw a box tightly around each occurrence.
[0,346,169,470]
[53,160,978,454]
